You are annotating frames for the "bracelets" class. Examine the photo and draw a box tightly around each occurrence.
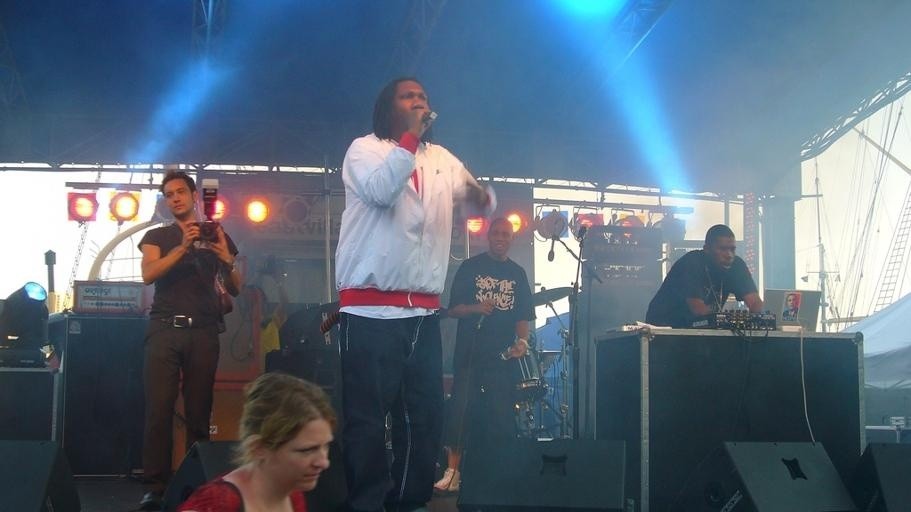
[223,258,236,273]
[519,338,528,348]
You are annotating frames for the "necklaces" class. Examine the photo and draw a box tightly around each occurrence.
[705,263,724,312]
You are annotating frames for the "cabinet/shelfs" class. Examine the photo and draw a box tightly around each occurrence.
[590,328,866,511]
[48,312,150,475]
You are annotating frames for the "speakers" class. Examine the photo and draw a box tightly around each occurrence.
[151,438,356,511]
[454,435,629,512]
[664,440,863,511]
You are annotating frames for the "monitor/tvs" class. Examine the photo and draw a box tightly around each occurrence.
[186,177,223,240]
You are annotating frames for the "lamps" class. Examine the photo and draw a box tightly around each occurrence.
[466,197,694,245]
[65,181,311,226]
[0,283,50,368]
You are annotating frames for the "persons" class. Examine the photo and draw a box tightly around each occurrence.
[247,280,289,373]
[783,294,799,321]
[137,169,243,511]
[176,372,336,512]
[335,78,498,511]
[645,224,764,329]
[434,216,538,494]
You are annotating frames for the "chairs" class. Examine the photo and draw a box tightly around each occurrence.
[157,441,208,512]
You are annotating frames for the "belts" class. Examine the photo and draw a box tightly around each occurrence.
[150,311,216,329]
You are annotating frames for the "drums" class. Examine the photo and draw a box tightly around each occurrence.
[504,348,549,402]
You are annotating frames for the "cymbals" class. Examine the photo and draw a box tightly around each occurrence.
[531,286,572,307]
[537,349,562,357]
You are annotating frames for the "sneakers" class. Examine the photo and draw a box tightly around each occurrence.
[431,467,462,496]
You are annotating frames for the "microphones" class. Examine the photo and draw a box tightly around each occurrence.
[475,296,495,330]
[577,225,586,240]
[548,237,556,262]
[421,109,437,127]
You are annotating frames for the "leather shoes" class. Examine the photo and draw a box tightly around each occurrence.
[137,491,162,509]
